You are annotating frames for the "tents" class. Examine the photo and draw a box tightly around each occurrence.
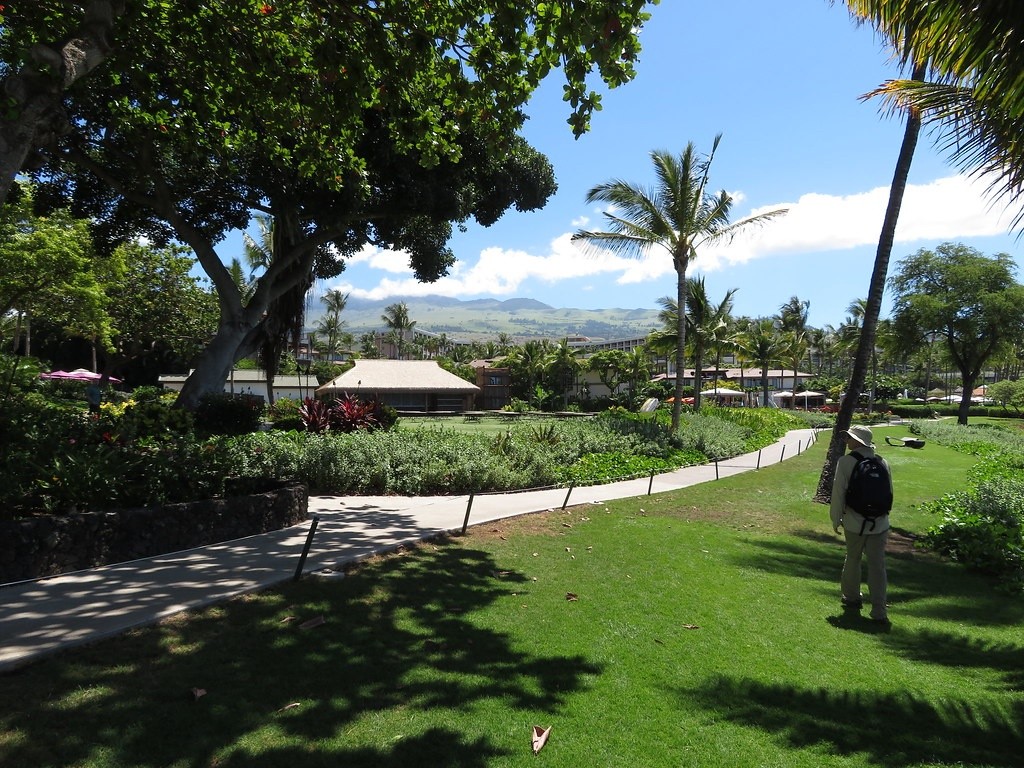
[770,391,796,407]
[795,389,826,411]
[700,387,749,407]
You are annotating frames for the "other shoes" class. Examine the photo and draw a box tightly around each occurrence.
[841,593,862,606]
[870,611,889,623]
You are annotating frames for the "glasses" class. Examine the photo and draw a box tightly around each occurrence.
[846,435,851,439]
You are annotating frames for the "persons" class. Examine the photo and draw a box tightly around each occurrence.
[829,423,894,625]
[85,379,103,415]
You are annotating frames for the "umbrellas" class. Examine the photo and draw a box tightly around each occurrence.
[40,366,122,385]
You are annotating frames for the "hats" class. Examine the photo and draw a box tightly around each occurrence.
[839,424,877,449]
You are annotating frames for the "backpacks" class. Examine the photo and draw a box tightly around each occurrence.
[845,451,893,517]
